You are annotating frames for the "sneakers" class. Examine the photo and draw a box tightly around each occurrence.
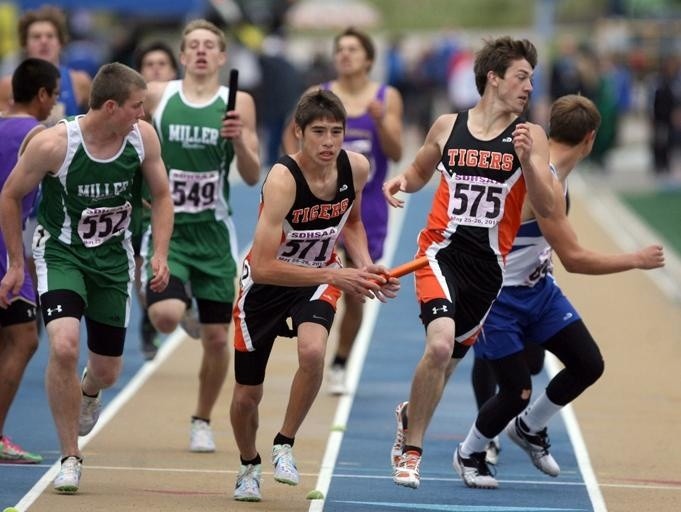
[51,455,82,491]
[506,416,561,477]
[0,435,43,465]
[138,319,160,362]
[486,440,501,464]
[77,366,102,436]
[452,442,499,489]
[234,463,264,502]
[271,444,299,485]
[391,401,408,468]
[188,419,216,453]
[393,450,423,489]
[325,366,346,395]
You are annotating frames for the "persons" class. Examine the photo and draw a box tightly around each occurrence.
[133,42,202,359]
[472,343,545,465]
[0,5,93,338]
[0,62,175,494]
[282,27,405,395]
[230,91,401,503]
[452,95,666,489]
[139,19,262,452]
[0,58,62,464]
[381,35,556,489]
